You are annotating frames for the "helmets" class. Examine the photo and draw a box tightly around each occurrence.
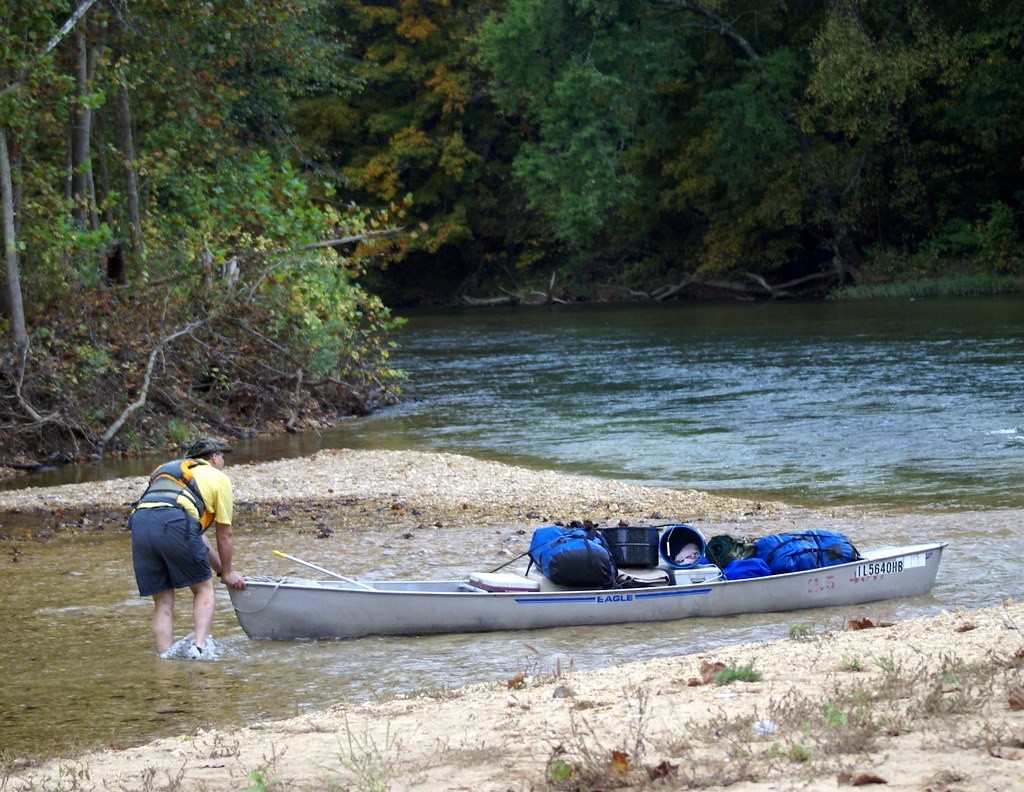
[184,438,234,458]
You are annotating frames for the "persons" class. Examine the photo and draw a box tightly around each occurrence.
[128,435,247,656]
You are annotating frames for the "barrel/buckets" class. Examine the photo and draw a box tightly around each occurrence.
[659,526,707,570]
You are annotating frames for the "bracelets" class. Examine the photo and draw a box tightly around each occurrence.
[217,570,223,577]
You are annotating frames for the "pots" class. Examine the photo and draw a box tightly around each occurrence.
[596,526,659,569]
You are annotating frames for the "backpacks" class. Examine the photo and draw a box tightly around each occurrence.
[530,524,617,589]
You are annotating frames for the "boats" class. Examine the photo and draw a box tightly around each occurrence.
[220,534,953,644]
